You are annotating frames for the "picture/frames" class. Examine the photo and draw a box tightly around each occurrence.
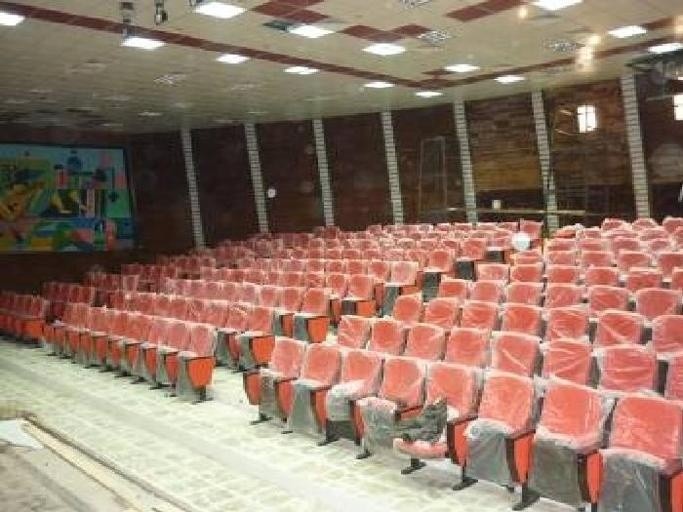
[0,140,139,258]
